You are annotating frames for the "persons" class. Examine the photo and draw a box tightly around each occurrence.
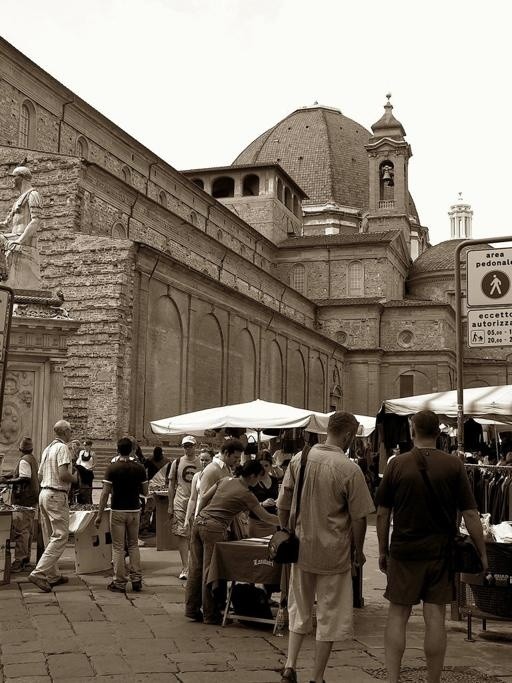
[271,411,376,683]
[0,436,39,573]
[125,436,301,580]
[376,409,489,683]
[183,459,281,625]
[0,166,43,291]
[27,419,77,592]
[94,438,148,593]
[68,439,96,504]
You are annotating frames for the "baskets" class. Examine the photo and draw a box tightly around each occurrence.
[469,584,512,617]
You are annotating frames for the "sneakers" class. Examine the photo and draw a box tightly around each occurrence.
[11,558,31,572]
[185,613,233,625]
[137,538,145,546]
[28,575,68,592]
[108,581,142,592]
[179,571,189,579]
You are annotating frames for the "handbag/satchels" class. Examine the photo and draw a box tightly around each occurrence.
[268,527,300,564]
[453,533,484,573]
[230,583,274,630]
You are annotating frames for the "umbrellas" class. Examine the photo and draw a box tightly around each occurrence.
[150,399,364,458]
[377,384,512,464]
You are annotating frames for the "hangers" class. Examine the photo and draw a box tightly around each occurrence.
[482,465,512,486]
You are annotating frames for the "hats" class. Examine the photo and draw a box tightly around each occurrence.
[20,437,33,451]
[182,436,196,444]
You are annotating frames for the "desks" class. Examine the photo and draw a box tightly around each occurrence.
[67,508,114,576]
[205,534,291,636]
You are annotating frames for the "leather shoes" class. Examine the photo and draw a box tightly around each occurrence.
[282,668,297,683]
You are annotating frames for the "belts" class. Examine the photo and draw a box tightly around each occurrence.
[41,487,67,492]
